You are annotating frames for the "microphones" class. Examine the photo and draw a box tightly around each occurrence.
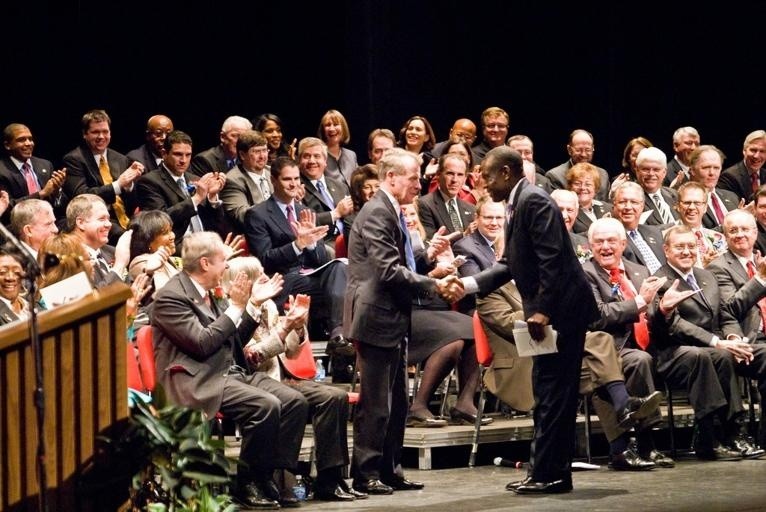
[493,456,524,468]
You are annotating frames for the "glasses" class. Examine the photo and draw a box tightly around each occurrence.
[680,201,705,205]
[570,145,595,153]
[482,125,509,128]
[478,215,504,221]
[453,129,476,139]
[520,151,533,155]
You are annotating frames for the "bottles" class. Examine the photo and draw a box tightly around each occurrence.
[292,475,306,501]
[314,359,325,383]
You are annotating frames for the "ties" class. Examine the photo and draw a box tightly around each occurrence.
[711,193,724,225]
[630,230,662,276]
[695,231,706,255]
[316,181,343,234]
[653,194,674,224]
[747,262,766,336]
[687,275,704,302]
[287,205,313,273]
[177,179,201,233]
[23,163,37,195]
[491,243,496,252]
[100,155,129,230]
[204,296,210,311]
[752,172,758,193]
[12,297,24,314]
[448,199,464,232]
[400,212,421,306]
[97,253,109,274]
[260,177,271,200]
[610,269,649,351]
[226,158,236,170]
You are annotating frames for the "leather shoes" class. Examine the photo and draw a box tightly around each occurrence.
[380,478,424,490]
[640,448,676,468]
[325,334,355,356]
[697,441,743,461]
[616,391,663,432]
[234,479,282,510]
[725,436,765,459]
[265,479,301,507]
[506,476,534,491]
[406,410,447,427]
[342,478,368,499]
[608,437,657,471]
[451,408,493,425]
[353,480,393,494]
[333,360,360,382]
[320,482,355,500]
[514,479,572,494]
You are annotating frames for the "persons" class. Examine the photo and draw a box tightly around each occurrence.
[0,106,465,510]
[413,106,765,494]
